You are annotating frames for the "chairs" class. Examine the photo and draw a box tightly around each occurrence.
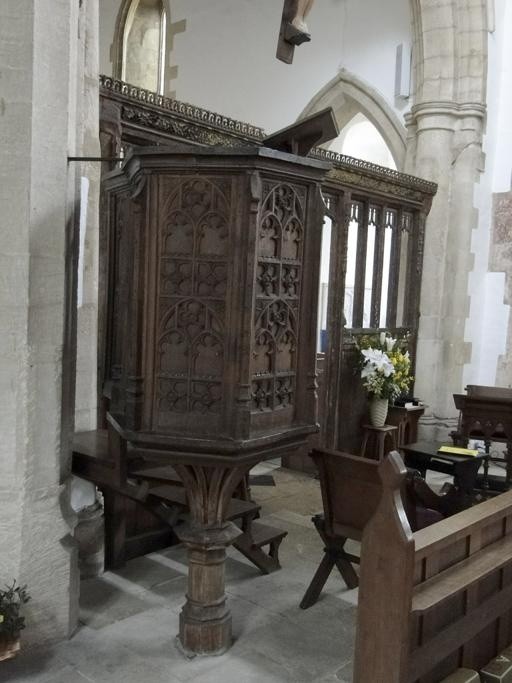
[452,381,512,492]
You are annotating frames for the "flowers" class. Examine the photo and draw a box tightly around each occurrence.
[351,325,417,407]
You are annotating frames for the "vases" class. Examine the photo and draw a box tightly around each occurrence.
[369,391,389,428]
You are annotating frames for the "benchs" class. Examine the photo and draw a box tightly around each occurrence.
[307,445,481,561]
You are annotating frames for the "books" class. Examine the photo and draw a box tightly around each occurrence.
[437,445,478,458]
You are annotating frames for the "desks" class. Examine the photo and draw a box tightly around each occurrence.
[396,438,490,508]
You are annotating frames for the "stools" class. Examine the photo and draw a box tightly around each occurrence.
[360,423,398,461]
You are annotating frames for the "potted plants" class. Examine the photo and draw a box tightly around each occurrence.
[1,577,33,664]
